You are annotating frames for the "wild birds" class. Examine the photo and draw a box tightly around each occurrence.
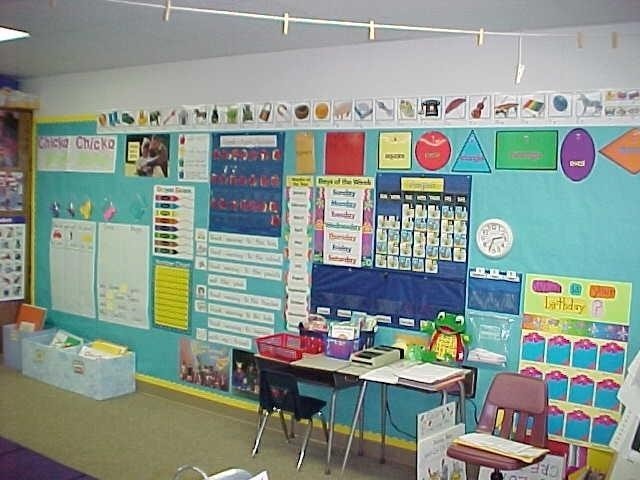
[354,106,373,119]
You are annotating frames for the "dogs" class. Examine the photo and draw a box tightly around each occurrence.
[577,93,601,115]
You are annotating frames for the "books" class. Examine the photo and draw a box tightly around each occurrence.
[394,359,474,391]
[327,312,379,361]
[16,302,128,359]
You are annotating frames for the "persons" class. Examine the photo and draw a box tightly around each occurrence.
[136,134,168,178]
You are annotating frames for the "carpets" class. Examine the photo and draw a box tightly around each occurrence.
[0,436,102,479]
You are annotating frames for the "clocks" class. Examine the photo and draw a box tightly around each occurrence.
[475,219,513,258]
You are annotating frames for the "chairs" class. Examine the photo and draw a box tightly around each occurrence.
[447,373,547,480]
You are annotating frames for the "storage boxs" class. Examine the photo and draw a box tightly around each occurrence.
[3,324,136,401]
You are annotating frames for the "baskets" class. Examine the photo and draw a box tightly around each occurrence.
[324,330,375,360]
[255,333,324,362]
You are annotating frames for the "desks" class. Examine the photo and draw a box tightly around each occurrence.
[254,351,471,476]
